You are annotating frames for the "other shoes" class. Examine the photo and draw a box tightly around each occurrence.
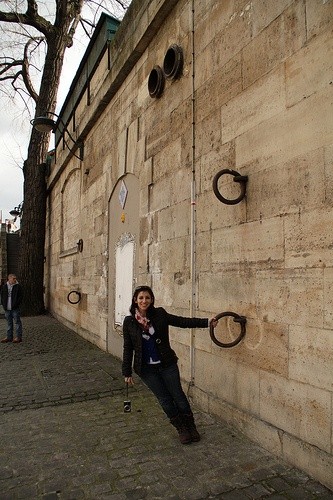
[12,336,22,343]
[1,337,11,343]
[178,431,191,444]
[189,429,200,442]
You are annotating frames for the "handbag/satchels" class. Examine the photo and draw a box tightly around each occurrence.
[156,339,178,368]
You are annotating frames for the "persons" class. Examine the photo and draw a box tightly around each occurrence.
[0,274,23,343]
[122,286,219,444]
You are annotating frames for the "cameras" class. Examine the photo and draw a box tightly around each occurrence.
[124,401,131,413]
